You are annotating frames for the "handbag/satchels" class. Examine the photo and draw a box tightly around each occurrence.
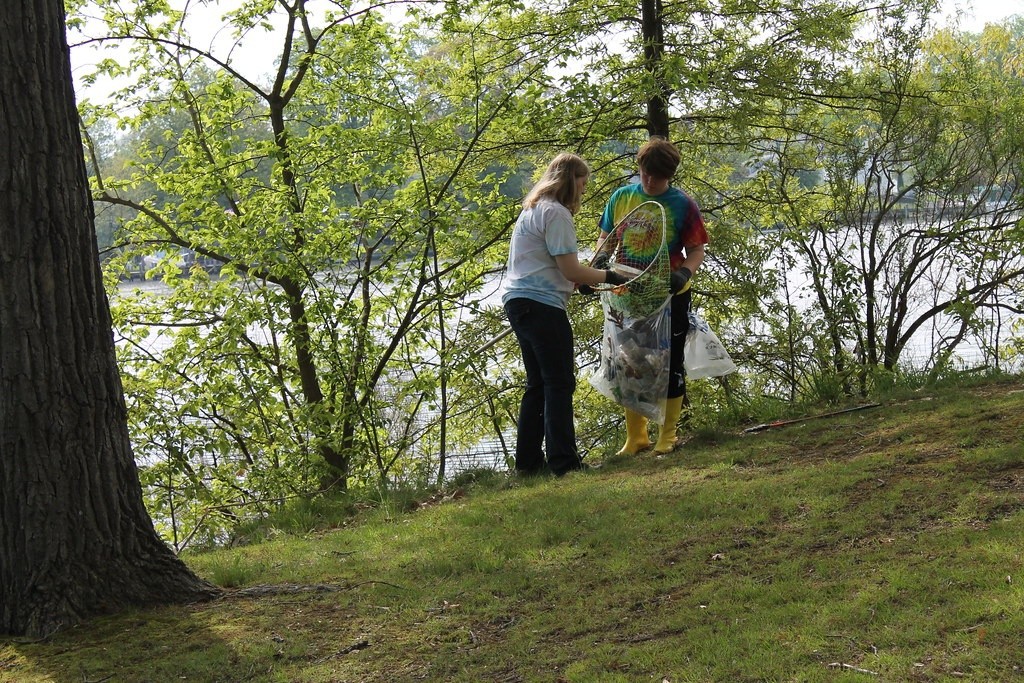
[682,310,739,381]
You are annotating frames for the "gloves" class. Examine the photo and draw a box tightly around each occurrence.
[604,270,631,288]
[592,250,609,269]
[668,267,692,295]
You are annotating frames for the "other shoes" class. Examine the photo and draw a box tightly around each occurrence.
[582,462,602,472]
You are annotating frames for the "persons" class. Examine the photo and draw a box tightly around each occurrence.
[577,139,710,456]
[500,152,631,476]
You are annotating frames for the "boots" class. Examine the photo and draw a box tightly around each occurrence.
[615,408,651,456]
[648,395,683,457]
